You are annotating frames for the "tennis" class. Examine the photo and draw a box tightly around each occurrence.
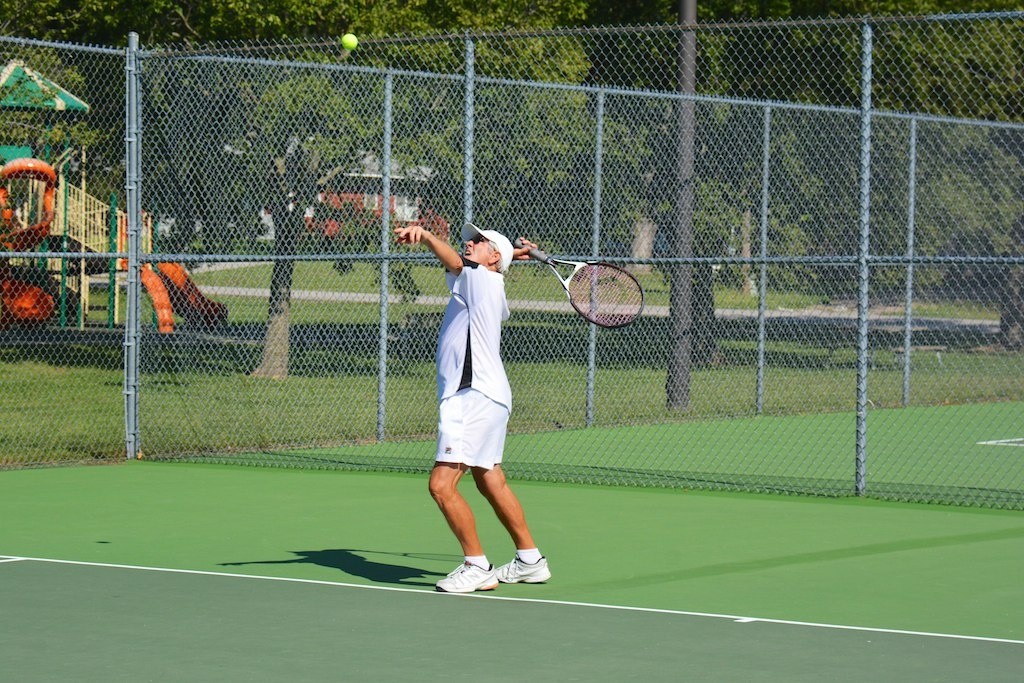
[341,33,358,51]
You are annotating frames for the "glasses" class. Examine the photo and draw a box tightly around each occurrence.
[467,237,485,244]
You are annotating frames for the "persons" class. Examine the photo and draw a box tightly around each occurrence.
[394,223,552,594]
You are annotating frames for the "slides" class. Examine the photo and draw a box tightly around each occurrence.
[122,259,229,335]
[0,158,55,324]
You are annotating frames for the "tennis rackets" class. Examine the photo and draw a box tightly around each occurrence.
[514,236,646,329]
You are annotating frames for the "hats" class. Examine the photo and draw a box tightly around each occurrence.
[461,222,514,270]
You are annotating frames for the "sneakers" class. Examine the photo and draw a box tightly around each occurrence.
[495,555,552,584]
[435,562,498,592]
